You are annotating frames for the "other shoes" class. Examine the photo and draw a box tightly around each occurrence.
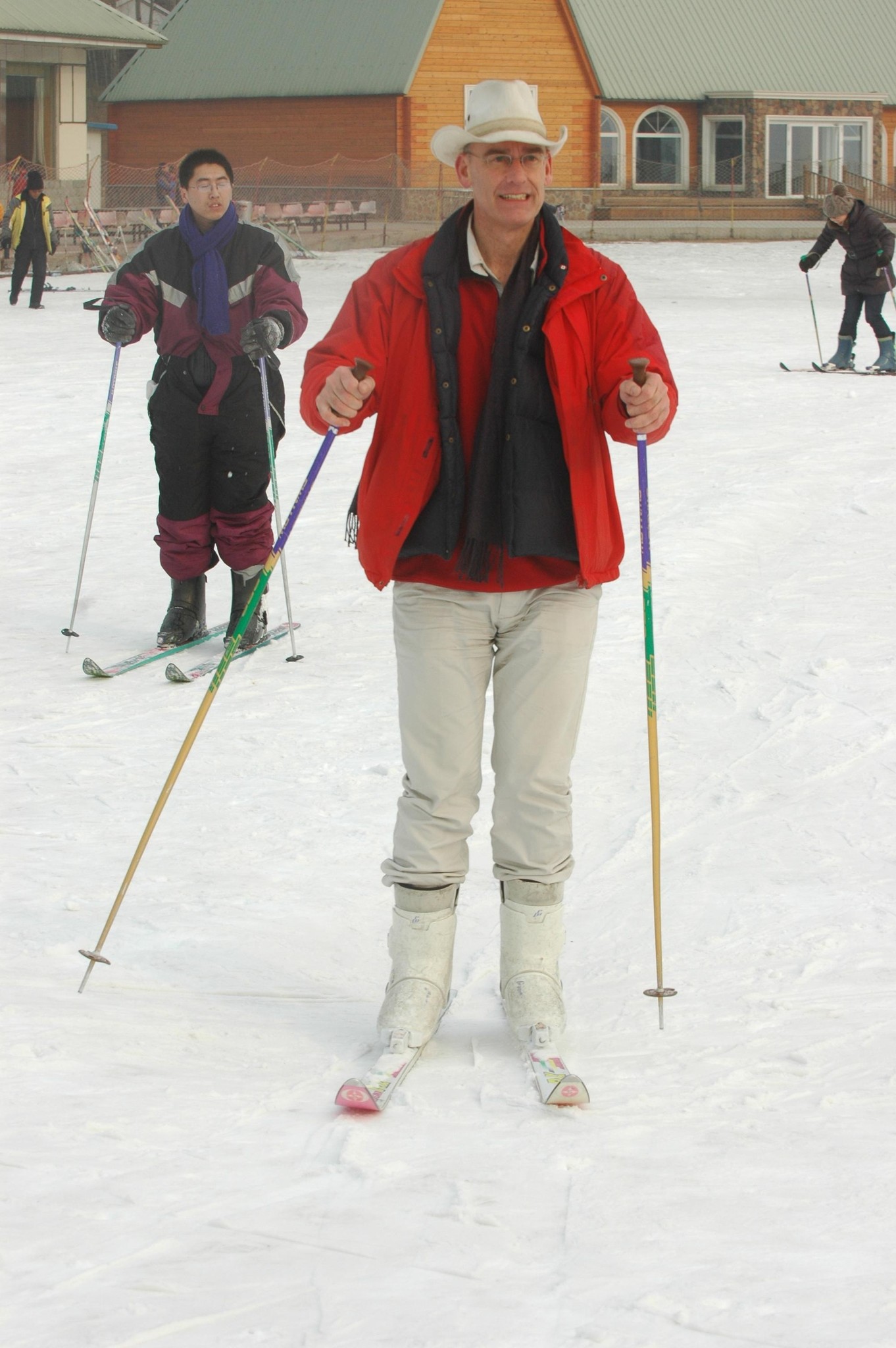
[10,294,17,306]
[29,305,44,309]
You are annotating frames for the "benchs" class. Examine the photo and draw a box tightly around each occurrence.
[53,200,378,244]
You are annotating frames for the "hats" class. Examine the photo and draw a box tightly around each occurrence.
[27,171,44,190]
[430,79,567,170]
[823,184,854,217]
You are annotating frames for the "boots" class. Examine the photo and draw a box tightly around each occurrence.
[224,569,269,650]
[500,879,566,1041]
[377,883,455,1048]
[157,574,207,648]
[866,332,896,372]
[823,335,856,371]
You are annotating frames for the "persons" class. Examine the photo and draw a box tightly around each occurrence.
[96,147,308,654]
[0,170,58,310]
[799,181,896,375]
[298,80,680,1111]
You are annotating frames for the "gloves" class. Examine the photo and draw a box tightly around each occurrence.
[49,241,56,255]
[239,316,284,360]
[874,254,891,268]
[799,257,814,273]
[101,304,137,344]
[1,238,11,249]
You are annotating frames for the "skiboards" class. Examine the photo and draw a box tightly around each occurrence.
[81,620,302,684]
[137,194,181,234]
[778,361,896,376]
[267,220,320,260]
[331,984,593,1114]
[64,195,121,272]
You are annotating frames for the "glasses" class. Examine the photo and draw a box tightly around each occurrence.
[462,153,548,170]
[186,182,232,193]
